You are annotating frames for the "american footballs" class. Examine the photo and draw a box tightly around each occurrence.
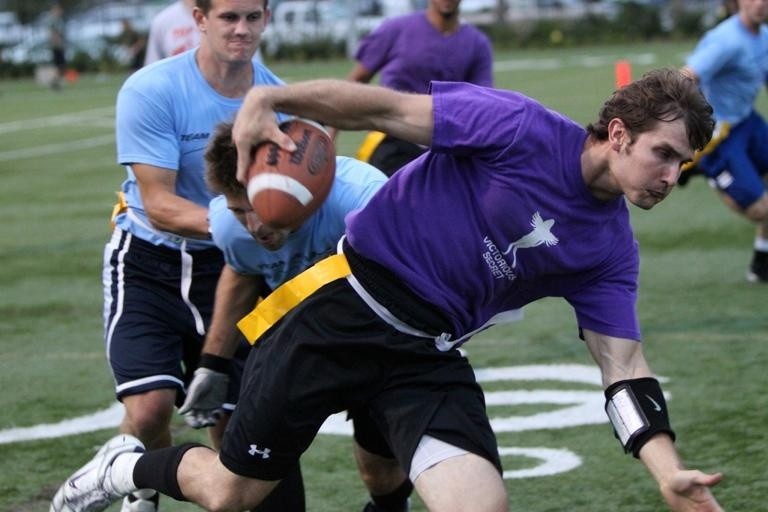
[247,117,336,229]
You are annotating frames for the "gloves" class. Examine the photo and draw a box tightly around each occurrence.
[177,366,231,429]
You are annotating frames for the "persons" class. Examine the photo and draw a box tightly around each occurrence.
[0,0,144,92]
[676,0,768,282]
[348,1,493,97]
[175,121,416,512]
[99,0,291,512]
[44,67,729,512]
[141,0,264,66]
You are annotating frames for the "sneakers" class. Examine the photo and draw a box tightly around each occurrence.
[48,434,147,511]
